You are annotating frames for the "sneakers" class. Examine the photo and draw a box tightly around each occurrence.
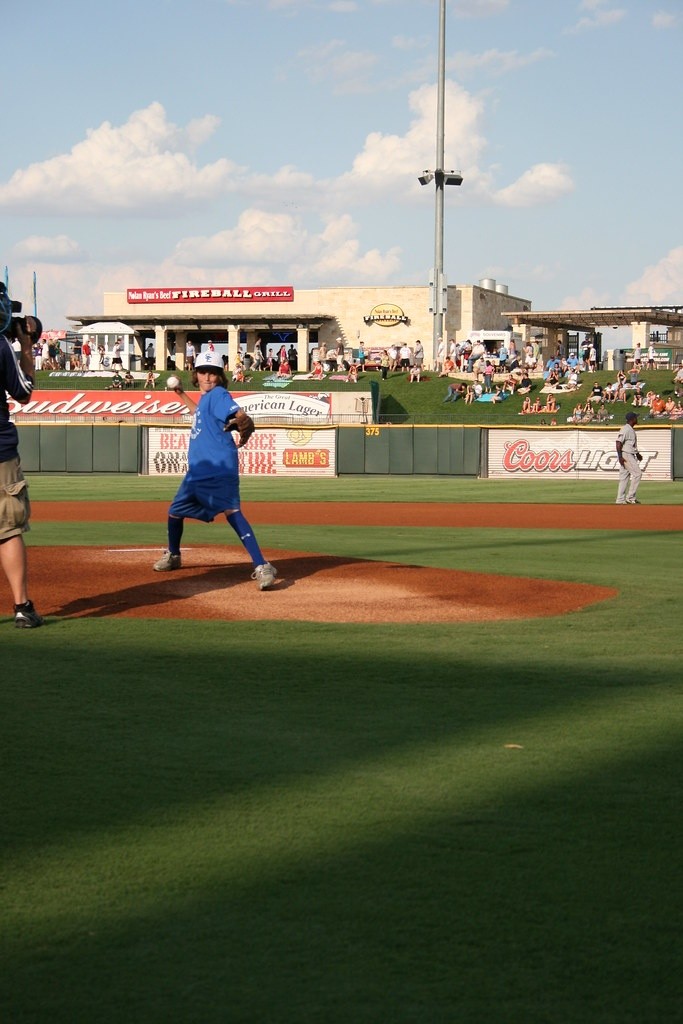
[616,497,641,505]
[13,600,43,629]
[251,562,278,590]
[153,550,181,572]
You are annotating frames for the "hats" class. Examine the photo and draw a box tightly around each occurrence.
[125,371,130,374]
[195,351,224,369]
[626,412,640,420]
[570,353,574,356]
[208,340,212,342]
[656,394,661,399]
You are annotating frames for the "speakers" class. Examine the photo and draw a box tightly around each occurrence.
[443,173,463,186]
[418,175,434,185]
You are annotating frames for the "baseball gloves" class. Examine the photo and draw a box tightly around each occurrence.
[222,414,255,449]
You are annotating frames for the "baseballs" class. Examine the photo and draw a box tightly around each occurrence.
[167,376,180,388]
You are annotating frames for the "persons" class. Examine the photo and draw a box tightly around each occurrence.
[0,281,46,628]
[375,340,424,383]
[153,350,277,591]
[308,337,367,383]
[435,336,683,426]
[616,411,643,504]
[11,335,105,371]
[112,338,155,390]
[167,340,228,371]
[232,337,298,384]
